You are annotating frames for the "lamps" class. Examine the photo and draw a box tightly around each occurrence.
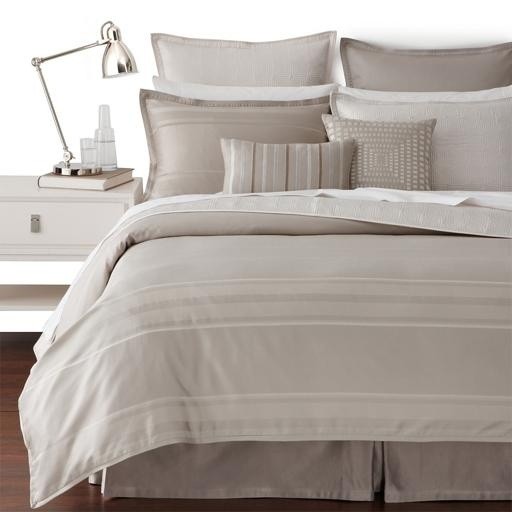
[31,20,139,175]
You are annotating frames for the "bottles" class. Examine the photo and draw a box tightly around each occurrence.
[95,105,116,171]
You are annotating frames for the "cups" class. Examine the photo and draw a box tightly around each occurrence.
[79,138,95,164]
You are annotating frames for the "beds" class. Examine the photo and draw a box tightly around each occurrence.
[85,187,512,510]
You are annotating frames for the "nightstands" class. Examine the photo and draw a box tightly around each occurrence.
[0,177,144,333]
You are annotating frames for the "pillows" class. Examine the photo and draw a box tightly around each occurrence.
[334,84,511,102]
[217,135,360,199]
[339,33,512,91]
[151,72,338,103]
[138,86,328,198]
[149,28,338,89]
[318,113,440,193]
[328,89,511,194]
